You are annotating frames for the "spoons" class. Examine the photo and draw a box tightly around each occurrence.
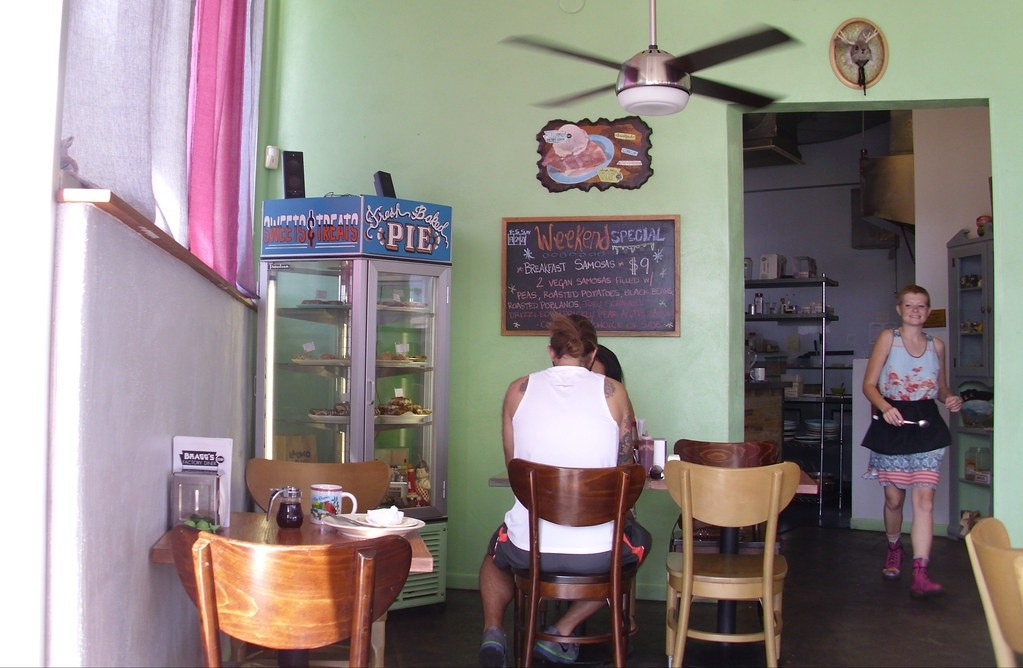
[899,419,929,429]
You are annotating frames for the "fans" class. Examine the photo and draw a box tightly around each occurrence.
[499,0,802,111]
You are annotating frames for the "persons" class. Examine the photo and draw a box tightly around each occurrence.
[480,314,652,668]
[860,286,963,600]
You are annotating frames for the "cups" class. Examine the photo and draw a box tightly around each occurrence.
[310,483,357,524]
[750,368,765,381]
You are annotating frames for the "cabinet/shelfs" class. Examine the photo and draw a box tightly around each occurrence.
[947,237,993,539]
[743,276,839,395]
[774,396,853,525]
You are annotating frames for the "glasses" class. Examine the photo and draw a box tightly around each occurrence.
[649,465,663,480]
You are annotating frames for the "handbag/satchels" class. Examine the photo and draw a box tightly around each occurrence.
[677,513,769,542]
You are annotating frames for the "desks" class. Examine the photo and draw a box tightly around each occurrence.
[489,468,818,668]
[151,512,434,668]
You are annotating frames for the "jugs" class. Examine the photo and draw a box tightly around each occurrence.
[267,486,303,529]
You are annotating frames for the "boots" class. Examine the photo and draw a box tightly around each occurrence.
[912,558,942,597]
[883,537,904,579]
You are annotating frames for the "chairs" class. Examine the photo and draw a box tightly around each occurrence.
[229,457,393,666]
[172,525,413,668]
[664,461,800,668]
[669,439,783,554]
[509,458,646,668]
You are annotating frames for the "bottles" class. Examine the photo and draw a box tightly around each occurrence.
[749,304,754,315]
[639,430,654,476]
[754,293,763,315]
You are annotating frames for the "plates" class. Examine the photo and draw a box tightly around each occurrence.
[322,512,425,537]
[296,304,349,308]
[380,415,428,424]
[377,360,426,367]
[307,413,349,423]
[292,359,351,365]
[380,307,427,311]
[784,419,839,442]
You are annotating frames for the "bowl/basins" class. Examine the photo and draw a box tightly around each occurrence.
[831,388,845,395]
[366,509,404,524]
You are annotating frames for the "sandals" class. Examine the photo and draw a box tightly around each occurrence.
[630,618,638,637]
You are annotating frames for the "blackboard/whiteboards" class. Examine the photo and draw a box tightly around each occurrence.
[502,214,681,337]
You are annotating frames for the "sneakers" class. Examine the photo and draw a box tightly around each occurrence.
[479,625,506,668]
[536,625,579,663]
